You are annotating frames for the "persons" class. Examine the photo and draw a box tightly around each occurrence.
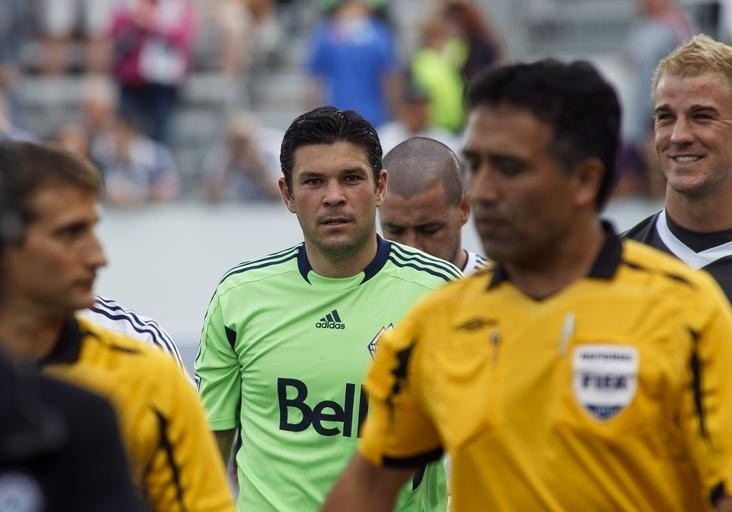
[617,33,732,307]
[378,136,491,278]
[75,294,191,381]
[194,106,467,511]
[0,140,140,512]
[32,312,236,511]
[320,59,732,512]
